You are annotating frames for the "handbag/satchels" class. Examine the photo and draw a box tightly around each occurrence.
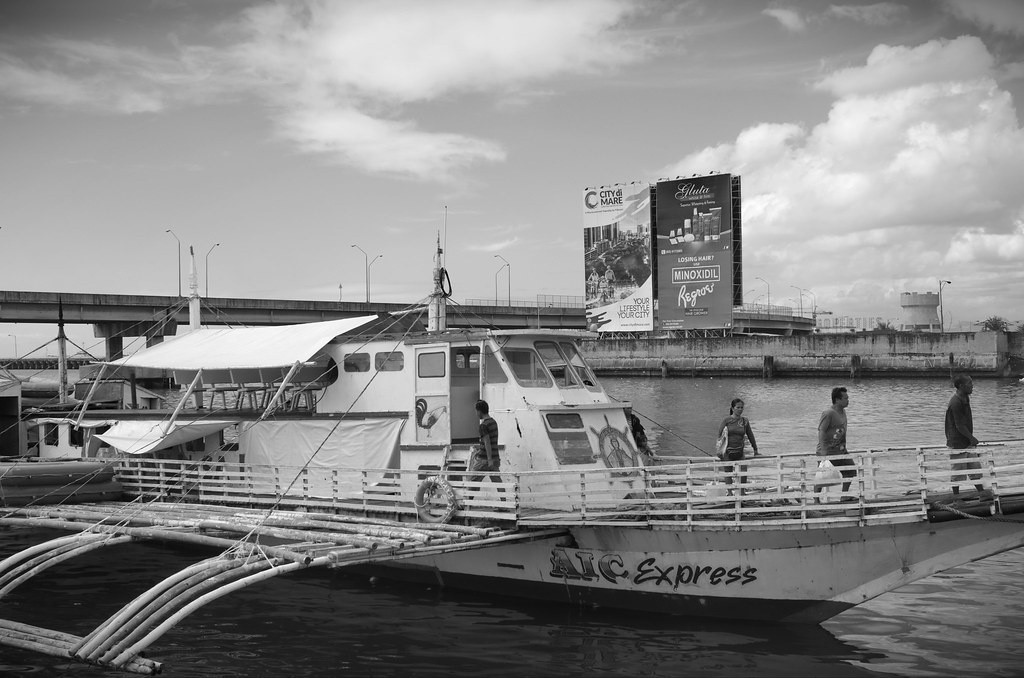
[815,459,843,488]
[717,422,728,457]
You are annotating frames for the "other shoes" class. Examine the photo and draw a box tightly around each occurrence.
[726,489,733,496]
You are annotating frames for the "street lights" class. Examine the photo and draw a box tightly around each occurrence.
[789,299,799,316]
[368,254,383,304]
[495,255,511,306]
[802,288,816,317]
[166,230,182,297]
[939,280,951,332]
[495,264,510,306]
[790,285,803,317]
[205,243,219,299]
[755,276,770,315]
[351,244,368,303]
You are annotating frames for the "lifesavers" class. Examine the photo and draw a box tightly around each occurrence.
[410,474,459,529]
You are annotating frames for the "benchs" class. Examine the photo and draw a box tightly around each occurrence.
[173,368,329,412]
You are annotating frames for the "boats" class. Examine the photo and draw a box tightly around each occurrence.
[0,205,1023,628]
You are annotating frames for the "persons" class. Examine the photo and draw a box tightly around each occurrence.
[587,265,615,297]
[945,373,1001,505]
[457,399,508,512]
[813,387,857,504]
[718,399,762,497]
